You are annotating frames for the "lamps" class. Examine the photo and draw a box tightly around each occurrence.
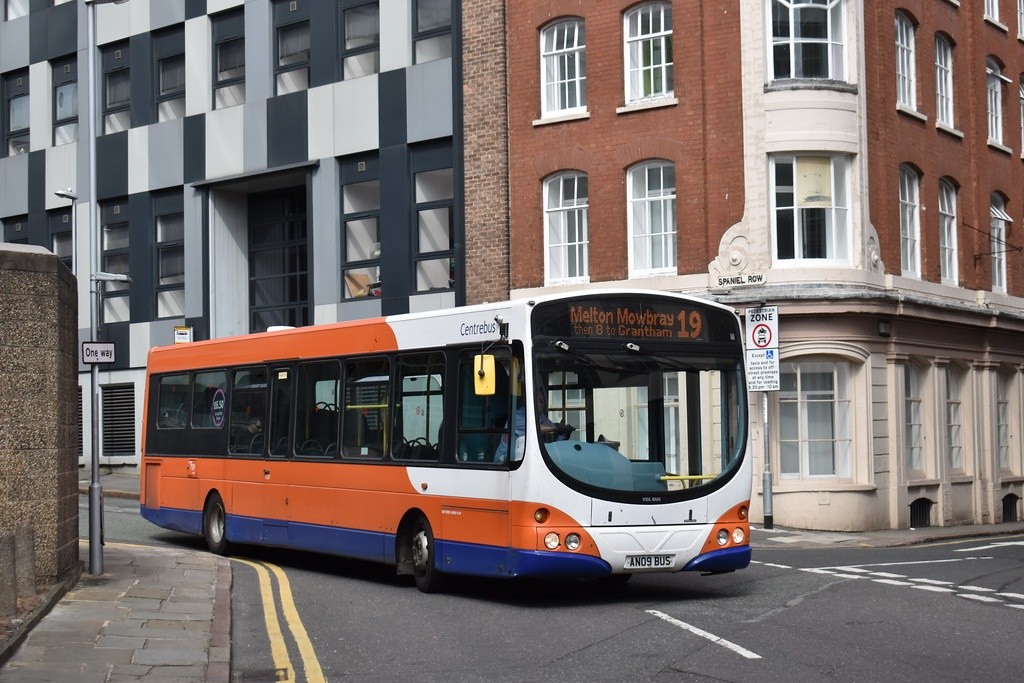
[369,242,382,283]
[877,316,889,336]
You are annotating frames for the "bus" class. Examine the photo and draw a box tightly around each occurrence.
[139,291,754,597]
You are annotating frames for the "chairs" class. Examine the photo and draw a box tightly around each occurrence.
[159,395,439,461]
[460,382,492,451]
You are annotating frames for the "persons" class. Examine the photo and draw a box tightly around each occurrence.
[494,391,574,464]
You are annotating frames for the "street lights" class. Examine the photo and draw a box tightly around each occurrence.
[53,188,78,277]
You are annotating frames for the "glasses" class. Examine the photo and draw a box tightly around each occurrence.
[254,424,261,429]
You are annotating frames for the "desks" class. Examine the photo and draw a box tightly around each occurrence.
[367,282,381,296]
